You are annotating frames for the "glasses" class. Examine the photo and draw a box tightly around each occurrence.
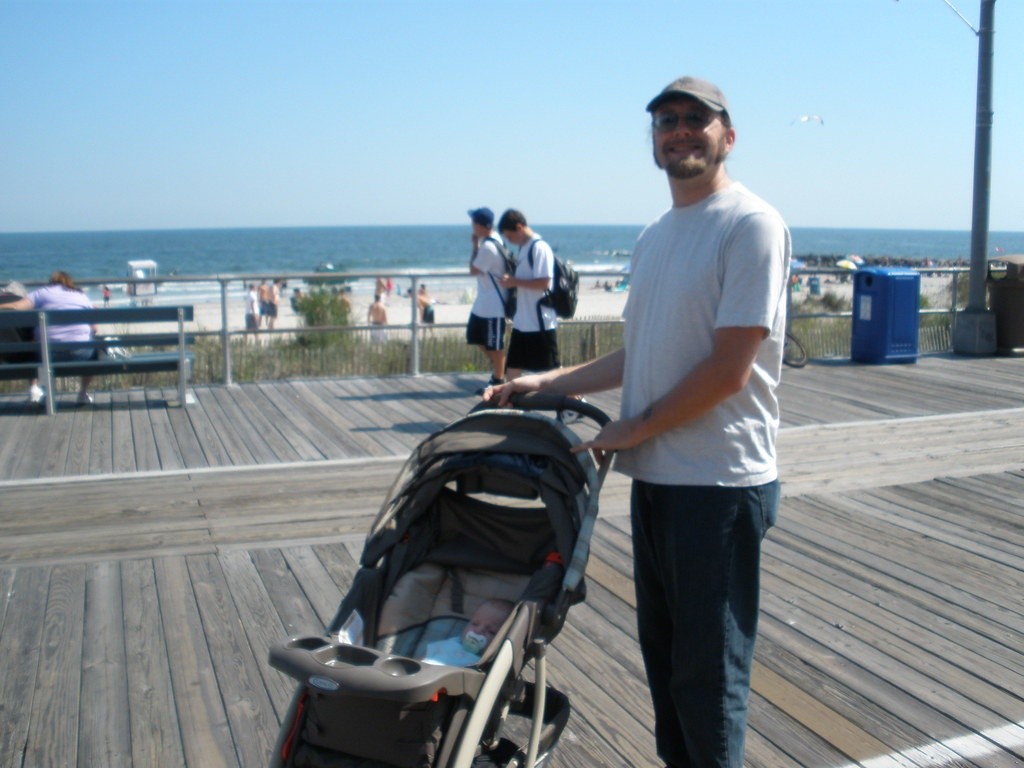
[651,113,726,129]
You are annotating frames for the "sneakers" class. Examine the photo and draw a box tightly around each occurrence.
[26,387,46,408]
[74,390,93,405]
[474,375,504,396]
[559,396,589,424]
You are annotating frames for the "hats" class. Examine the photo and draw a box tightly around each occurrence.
[645,76,733,126]
[467,206,494,227]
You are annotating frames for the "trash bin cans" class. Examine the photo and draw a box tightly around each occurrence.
[851,265,920,365]
[987,255,1024,352]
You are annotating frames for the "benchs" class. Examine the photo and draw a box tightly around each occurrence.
[0,303,195,415]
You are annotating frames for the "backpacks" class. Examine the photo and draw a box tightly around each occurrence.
[518,238,579,320]
[480,237,518,321]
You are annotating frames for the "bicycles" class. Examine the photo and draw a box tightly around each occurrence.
[780,330,809,369]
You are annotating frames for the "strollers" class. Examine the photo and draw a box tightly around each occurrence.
[266,391,613,768]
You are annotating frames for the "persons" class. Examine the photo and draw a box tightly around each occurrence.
[103,286,110,306]
[481,76,793,768]
[425,595,519,669]
[241,278,435,341]
[0,269,101,406]
[465,206,590,424]
